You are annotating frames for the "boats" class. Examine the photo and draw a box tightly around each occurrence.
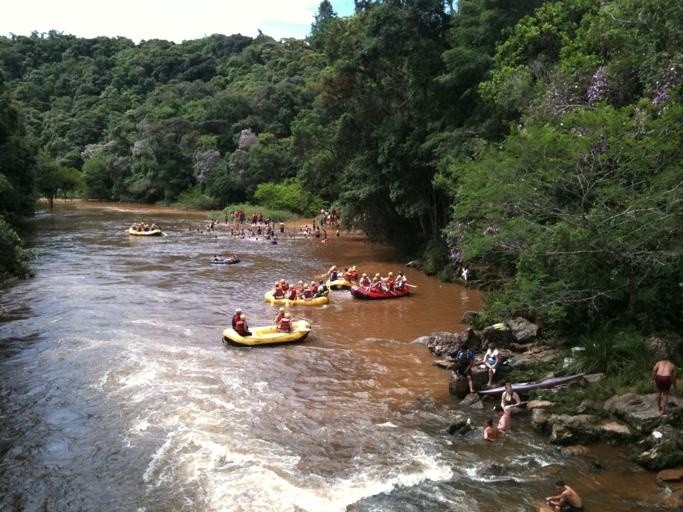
[129,224,162,237]
[264,290,329,307]
[351,284,409,299]
[210,257,240,263]
[478,373,583,398]
[223,320,311,347]
[325,273,359,290]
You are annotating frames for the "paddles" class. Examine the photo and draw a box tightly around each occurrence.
[286,297,290,307]
[270,294,276,307]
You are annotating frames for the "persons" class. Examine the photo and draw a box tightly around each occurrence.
[276,313,291,333]
[650,355,678,414]
[493,405,513,431]
[271,308,285,325]
[210,254,238,262]
[271,278,327,300]
[234,315,251,336]
[327,265,358,281]
[359,271,407,292]
[231,309,241,328]
[483,417,507,442]
[188,206,339,246]
[451,344,476,393]
[546,480,583,511]
[482,342,500,385]
[132,222,160,231]
[500,381,521,410]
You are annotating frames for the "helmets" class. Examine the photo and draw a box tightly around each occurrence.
[241,314,246,320]
[275,279,315,289]
[235,309,242,314]
[279,307,284,312]
[348,266,357,272]
[363,271,404,279]
[284,314,290,318]
[329,265,336,270]
